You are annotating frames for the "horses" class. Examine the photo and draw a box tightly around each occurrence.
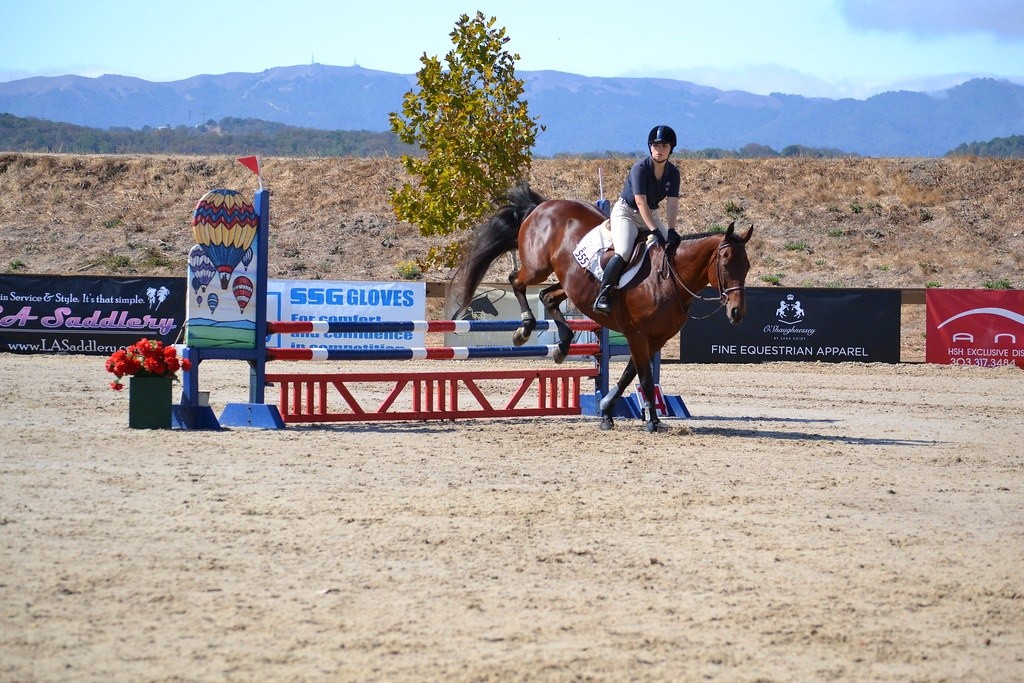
[442,181,755,433]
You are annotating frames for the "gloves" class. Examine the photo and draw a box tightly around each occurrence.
[651,227,666,251]
[667,228,680,243]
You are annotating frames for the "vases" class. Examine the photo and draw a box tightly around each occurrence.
[129,377,172,430]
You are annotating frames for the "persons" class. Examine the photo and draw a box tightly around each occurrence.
[593,126,680,314]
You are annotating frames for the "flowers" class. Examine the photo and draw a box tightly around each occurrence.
[105,338,192,390]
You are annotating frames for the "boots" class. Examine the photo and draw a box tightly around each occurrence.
[591,253,627,314]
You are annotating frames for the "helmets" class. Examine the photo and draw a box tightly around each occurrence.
[648,125,677,147]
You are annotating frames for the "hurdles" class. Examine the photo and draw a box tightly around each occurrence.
[165,188,696,432]
